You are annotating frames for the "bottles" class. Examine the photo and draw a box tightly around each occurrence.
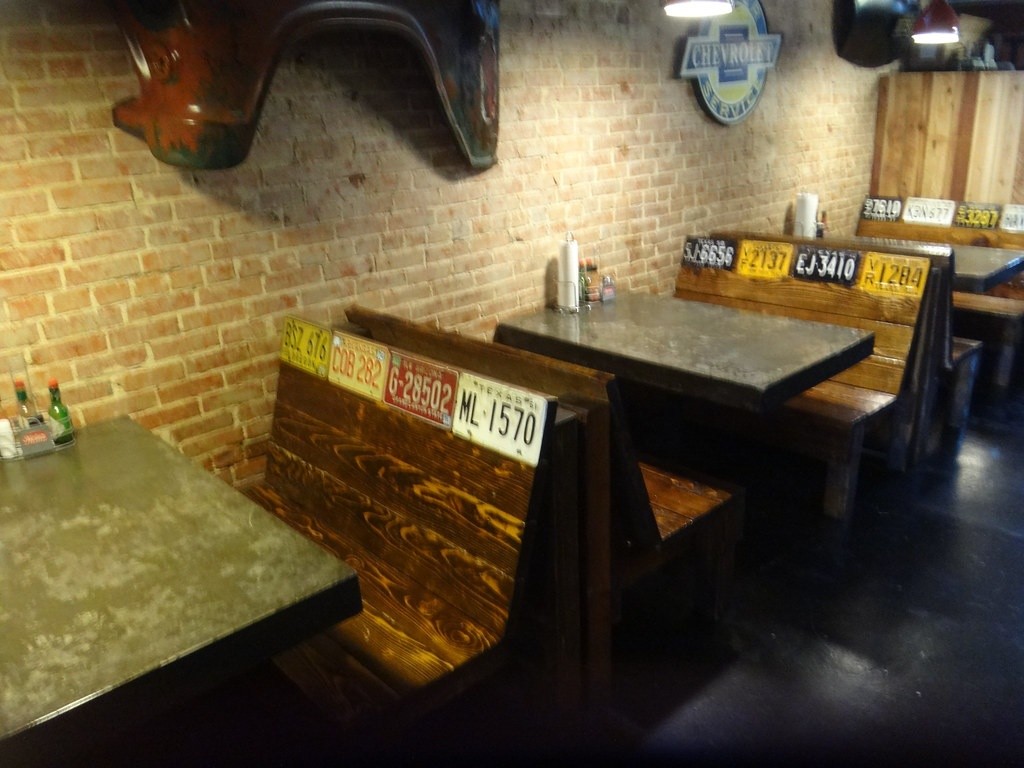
[579,261,615,302]
[14,380,37,421]
[48,378,74,443]
[821,211,827,237]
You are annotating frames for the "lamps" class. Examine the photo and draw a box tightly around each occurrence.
[910,0,962,44]
[660,0,734,17]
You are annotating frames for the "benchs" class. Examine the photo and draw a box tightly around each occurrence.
[241,310,560,768]
[855,195,1024,387]
[344,303,740,634]
[708,228,981,456]
[674,234,933,520]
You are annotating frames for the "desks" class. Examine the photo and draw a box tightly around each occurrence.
[0,411,363,768]
[498,292,876,417]
[852,235,1024,293]
